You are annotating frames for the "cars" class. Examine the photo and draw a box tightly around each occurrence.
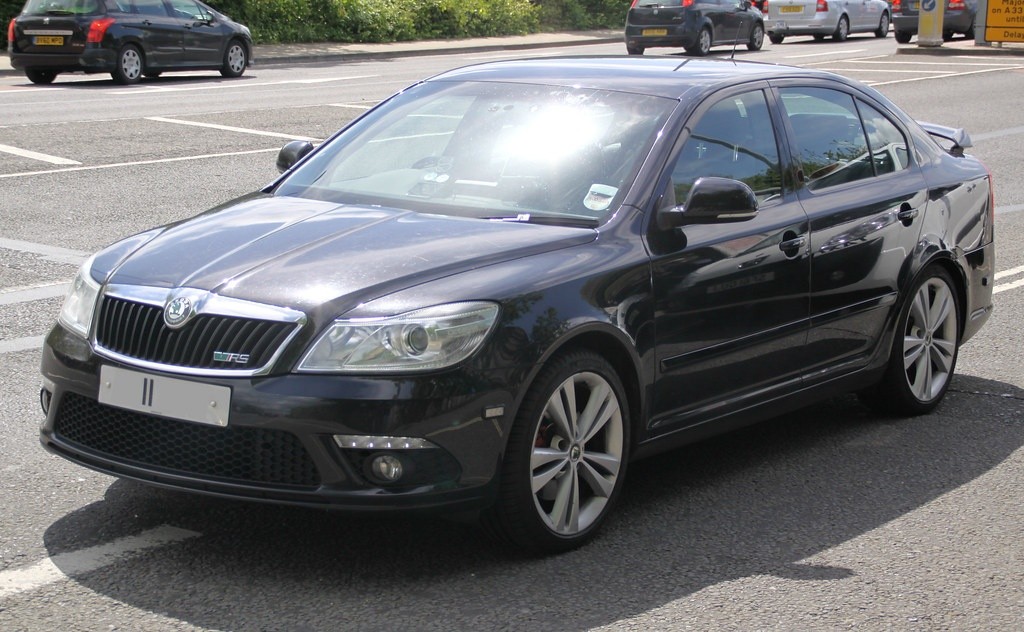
[8,0,255,80]
[763,0,892,40]
[34,53,998,551]
[625,0,764,55]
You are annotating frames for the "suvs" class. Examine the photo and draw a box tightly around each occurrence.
[891,1,977,44]
